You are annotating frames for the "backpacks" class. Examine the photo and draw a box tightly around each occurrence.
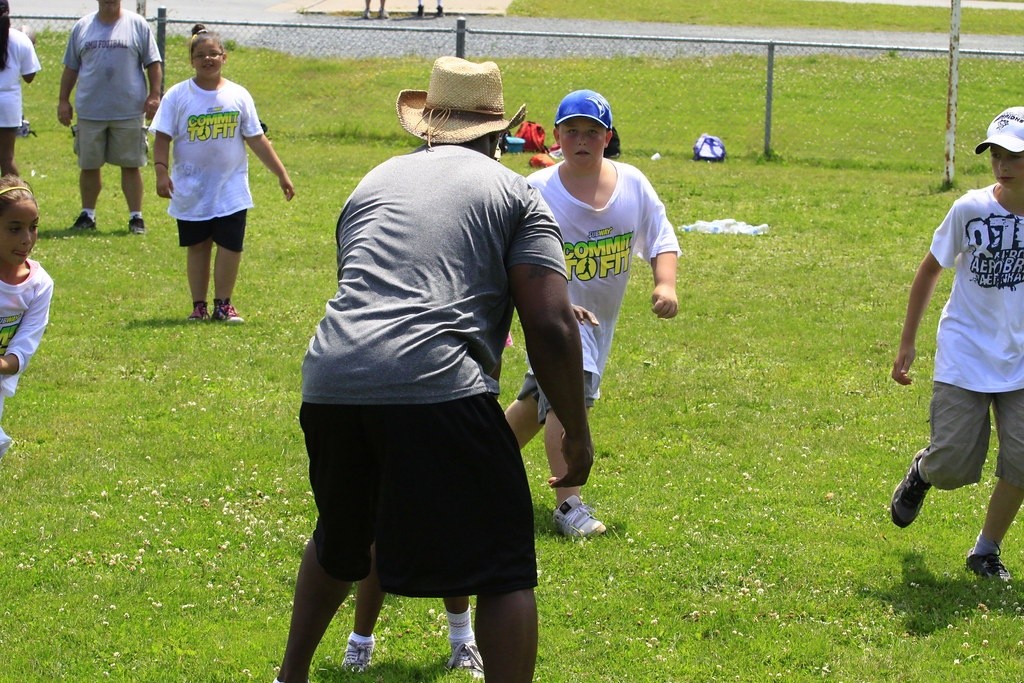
[694,134,725,160]
[516,120,549,153]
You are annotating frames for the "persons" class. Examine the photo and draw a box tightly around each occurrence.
[890,105,1024,582]
[274,55,596,683]
[0,175,54,458]
[57,0,163,236]
[148,24,294,324]
[344,544,484,678]
[0,0,41,177]
[363,0,390,19]
[417,0,444,18]
[505,89,681,540]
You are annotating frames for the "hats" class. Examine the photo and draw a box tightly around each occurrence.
[554,89,612,130]
[975,107,1024,155]
[396,56,526,147]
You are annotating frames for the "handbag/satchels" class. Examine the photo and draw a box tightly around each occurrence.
[604,127,621,157]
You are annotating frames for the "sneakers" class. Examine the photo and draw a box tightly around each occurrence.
[188,301,210,321]
[447,641,484,678]
[967,542,1010,581]
[212,298,244,324]
[554,495,606,540]
[70,211,97,231]
[341,639,374,672]
[127,216,144,234]
[890,448,931,528]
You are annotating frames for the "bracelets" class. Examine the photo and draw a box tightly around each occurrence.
[154,161,168,170]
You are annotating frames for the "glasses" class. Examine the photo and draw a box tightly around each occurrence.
[193,53,223,60]
